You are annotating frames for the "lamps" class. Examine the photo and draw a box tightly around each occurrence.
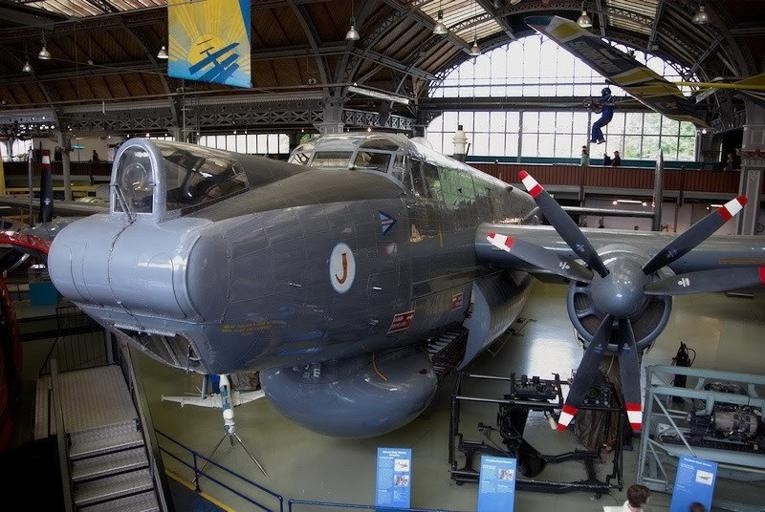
[156,33,169,61]
[577,7,594,28]
[36,11,53,62]
[25,44,32,74]
[691,4,711,27]
[469,19,482,58]
[346,3,361,42]
[431,3,447,35]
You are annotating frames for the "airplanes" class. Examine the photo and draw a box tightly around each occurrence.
[1,132,764,441]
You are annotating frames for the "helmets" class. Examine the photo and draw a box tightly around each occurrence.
[601,88,611,96]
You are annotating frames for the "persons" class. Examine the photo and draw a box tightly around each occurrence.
[93,149,99,160]
[620,483,653,511]
[609,150,621,165]
[587,85,616,143]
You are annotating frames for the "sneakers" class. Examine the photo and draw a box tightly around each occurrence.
[587,140,606,144]
